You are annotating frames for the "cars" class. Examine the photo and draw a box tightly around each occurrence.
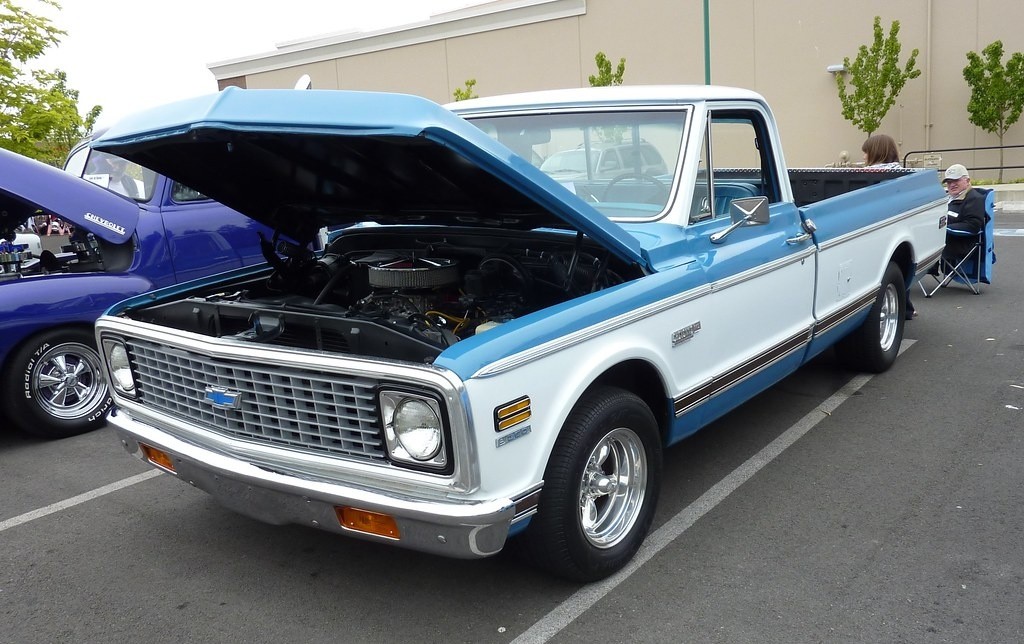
[0,128,317,435]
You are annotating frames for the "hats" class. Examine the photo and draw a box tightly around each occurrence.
[941,164,969,184]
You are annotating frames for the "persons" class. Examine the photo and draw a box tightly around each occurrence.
[860,133,919,321]
[928,164,986,275]
[89,158,140,200]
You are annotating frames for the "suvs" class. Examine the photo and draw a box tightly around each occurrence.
[539,138,670,196]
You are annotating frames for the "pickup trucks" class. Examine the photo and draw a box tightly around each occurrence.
[88,84,949,582]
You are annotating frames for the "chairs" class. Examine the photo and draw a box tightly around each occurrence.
[928,187,997,297]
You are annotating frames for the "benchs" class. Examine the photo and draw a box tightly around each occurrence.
[575,181,759,218]
